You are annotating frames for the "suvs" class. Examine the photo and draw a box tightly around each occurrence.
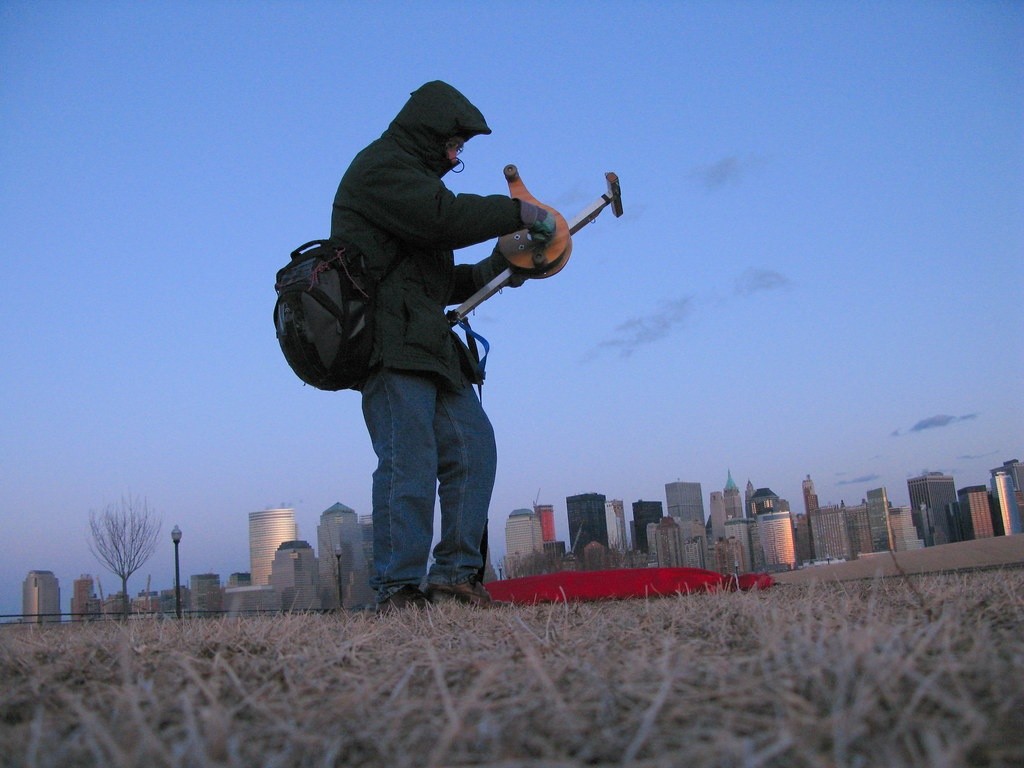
[564,524,584,561]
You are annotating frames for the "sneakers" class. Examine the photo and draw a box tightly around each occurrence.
[424,579,514,608]
[377,584,427,615]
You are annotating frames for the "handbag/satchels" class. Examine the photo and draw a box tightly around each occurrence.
[273,239,383,392]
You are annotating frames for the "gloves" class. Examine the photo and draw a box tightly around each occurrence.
[476,241,531,300]
[514,197,557,248]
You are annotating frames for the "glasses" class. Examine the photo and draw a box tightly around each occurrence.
[446,140,464,156]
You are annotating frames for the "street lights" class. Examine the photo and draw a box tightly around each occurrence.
[171,524,183,618]
[498,561,504,580]
[735,561,740,578]
[827,553,830,565]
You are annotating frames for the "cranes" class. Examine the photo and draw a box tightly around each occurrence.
[532,487,541,515]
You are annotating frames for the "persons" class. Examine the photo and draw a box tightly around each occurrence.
[330,80,557,616]
